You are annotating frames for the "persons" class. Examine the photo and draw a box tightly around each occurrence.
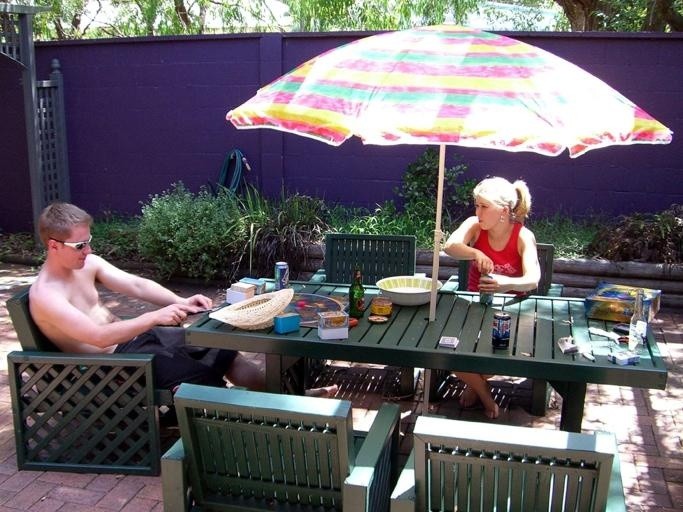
[26,202,339,403]
[439,173,542,419]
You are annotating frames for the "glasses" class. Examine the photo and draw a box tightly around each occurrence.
[47,235,93,251]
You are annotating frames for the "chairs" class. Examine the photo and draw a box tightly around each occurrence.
[384,404,631,512]
[2,274,179,478]
[424,238,558,419]
[156,377,406,511]
[285,228,423,406]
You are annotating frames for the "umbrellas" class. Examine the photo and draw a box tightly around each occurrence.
[226,18,676,423]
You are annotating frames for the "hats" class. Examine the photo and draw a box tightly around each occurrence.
[206,287,295,333]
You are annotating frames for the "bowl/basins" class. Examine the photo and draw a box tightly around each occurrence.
[376,276,443,307]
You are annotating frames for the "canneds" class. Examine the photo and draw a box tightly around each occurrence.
[274,262,290,291]
[491,311,511,349]
[480,272,495,304]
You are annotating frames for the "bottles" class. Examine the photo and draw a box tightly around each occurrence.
[628,289,648,356]
[348,266,364,319]
